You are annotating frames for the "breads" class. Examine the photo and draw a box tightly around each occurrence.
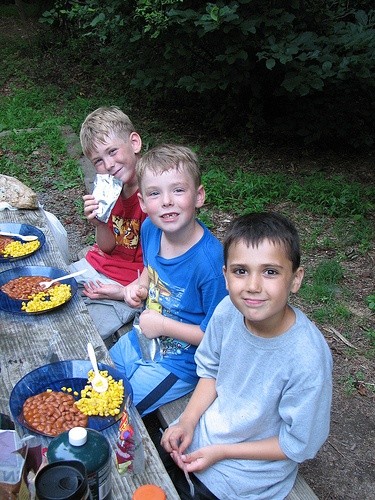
[0,174,38,209]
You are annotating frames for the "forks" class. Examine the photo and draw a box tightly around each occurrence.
[181,461,194,497]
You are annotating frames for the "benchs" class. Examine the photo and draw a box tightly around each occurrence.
[62,246,322,500]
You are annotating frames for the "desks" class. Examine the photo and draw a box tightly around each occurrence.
[0,208,179,500]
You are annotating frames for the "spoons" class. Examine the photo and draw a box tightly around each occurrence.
[38,268,87,288]
[0,232,38,242]
[87,343,109,392]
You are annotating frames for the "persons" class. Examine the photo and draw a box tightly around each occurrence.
[158,212,333,500]
[66,106,148,341]
[108,139,229,418]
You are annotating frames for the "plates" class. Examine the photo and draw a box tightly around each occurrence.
[9,359,133,438]
[0,265,78,316]
[0,222,45,262]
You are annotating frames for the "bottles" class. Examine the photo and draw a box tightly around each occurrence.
[32,460,92,500]
[47,426,113,500]
